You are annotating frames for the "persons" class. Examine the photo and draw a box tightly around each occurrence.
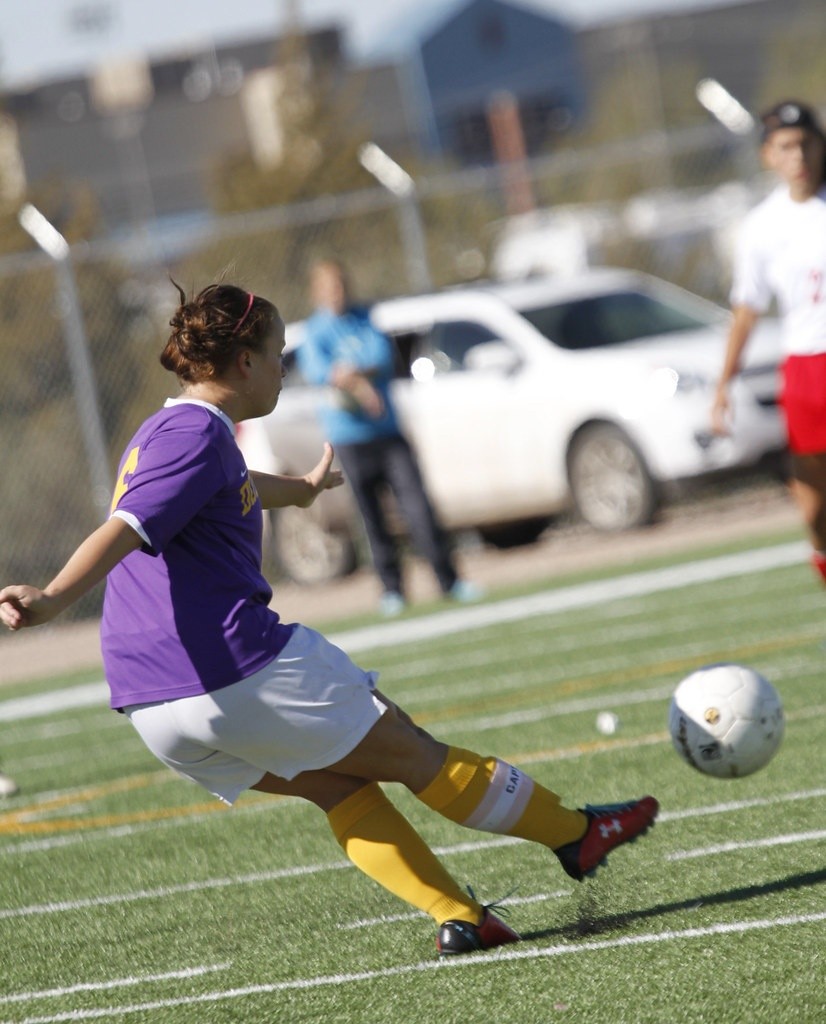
[294,259,483,621]
[707,95,825,590]
[0,277,656,955]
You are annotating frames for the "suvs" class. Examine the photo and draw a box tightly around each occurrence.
[226,261,789,592]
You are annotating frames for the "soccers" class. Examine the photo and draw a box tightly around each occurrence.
[667,661,786,779]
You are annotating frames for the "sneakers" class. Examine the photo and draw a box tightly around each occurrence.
[435,886,523,956]
[552,796,661,882]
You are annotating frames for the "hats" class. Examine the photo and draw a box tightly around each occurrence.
[760,100,817,130]
